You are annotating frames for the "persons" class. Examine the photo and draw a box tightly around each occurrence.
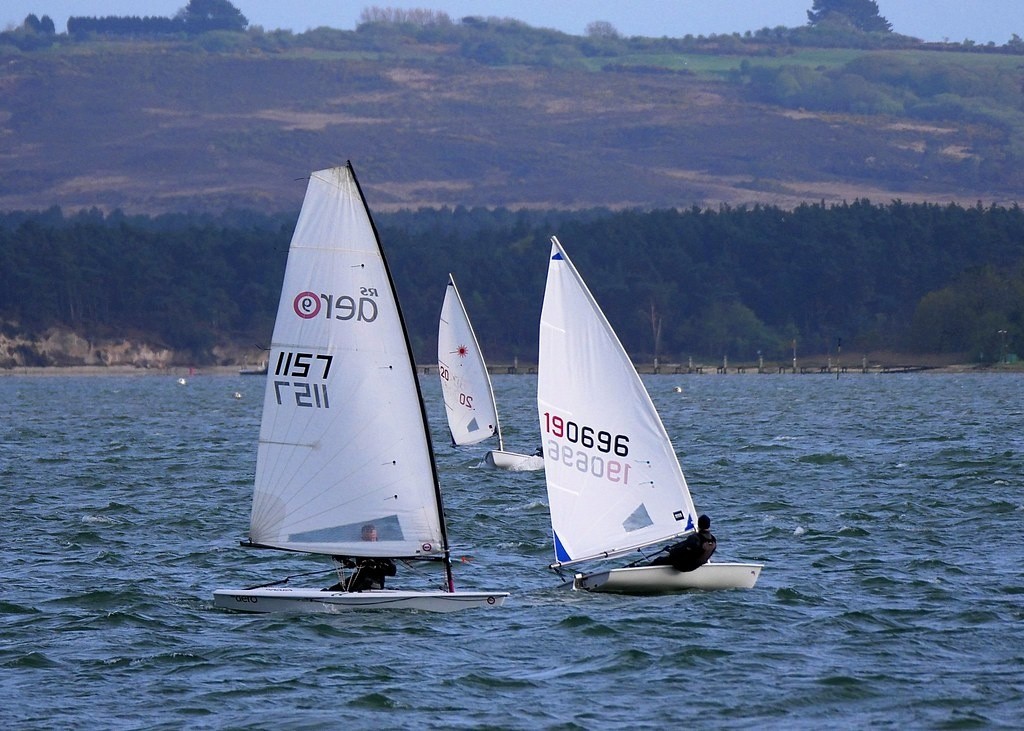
[631,514,717,572]
[320,525,396,593]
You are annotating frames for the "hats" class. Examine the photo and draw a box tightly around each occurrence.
[698,515,711,529]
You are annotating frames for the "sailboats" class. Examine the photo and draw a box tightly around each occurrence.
[438,272,544,471]
[536,235,764,591]
[213,158,510,613]
